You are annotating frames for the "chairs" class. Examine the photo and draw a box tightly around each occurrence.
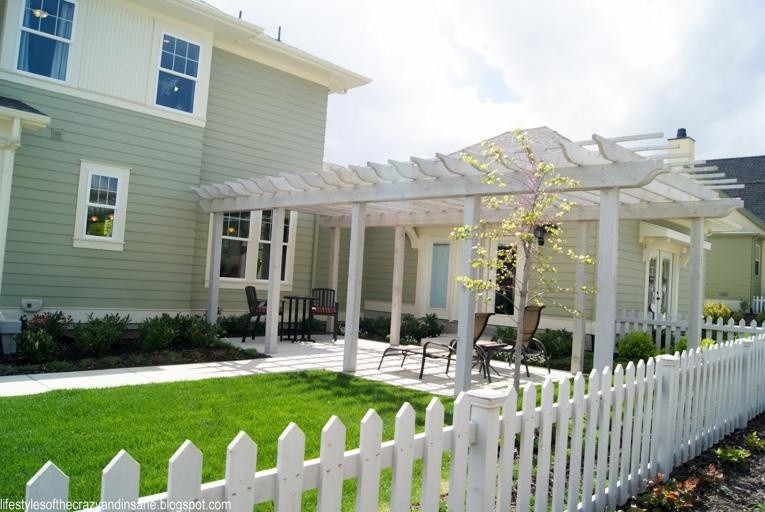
[243,286,339,342]
[378,305,551,379]
